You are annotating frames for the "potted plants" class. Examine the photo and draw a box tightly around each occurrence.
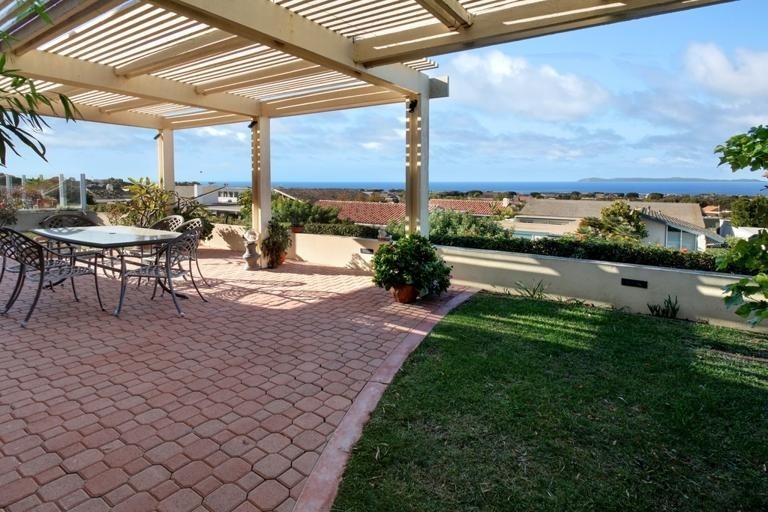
[369,231,453,303]
[104,176,215,255]
[260,219,292,269]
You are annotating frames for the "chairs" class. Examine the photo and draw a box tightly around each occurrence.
[0,213,210,328]
